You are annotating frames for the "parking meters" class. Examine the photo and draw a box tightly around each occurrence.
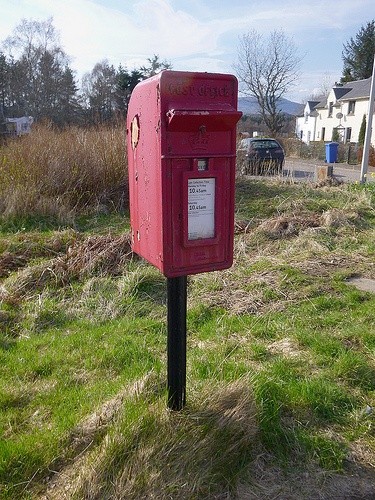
[123,70,243,276]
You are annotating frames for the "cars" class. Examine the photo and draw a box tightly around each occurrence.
[236,137,285,174]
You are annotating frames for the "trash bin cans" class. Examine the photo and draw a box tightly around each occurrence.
[324,142,339,163]
